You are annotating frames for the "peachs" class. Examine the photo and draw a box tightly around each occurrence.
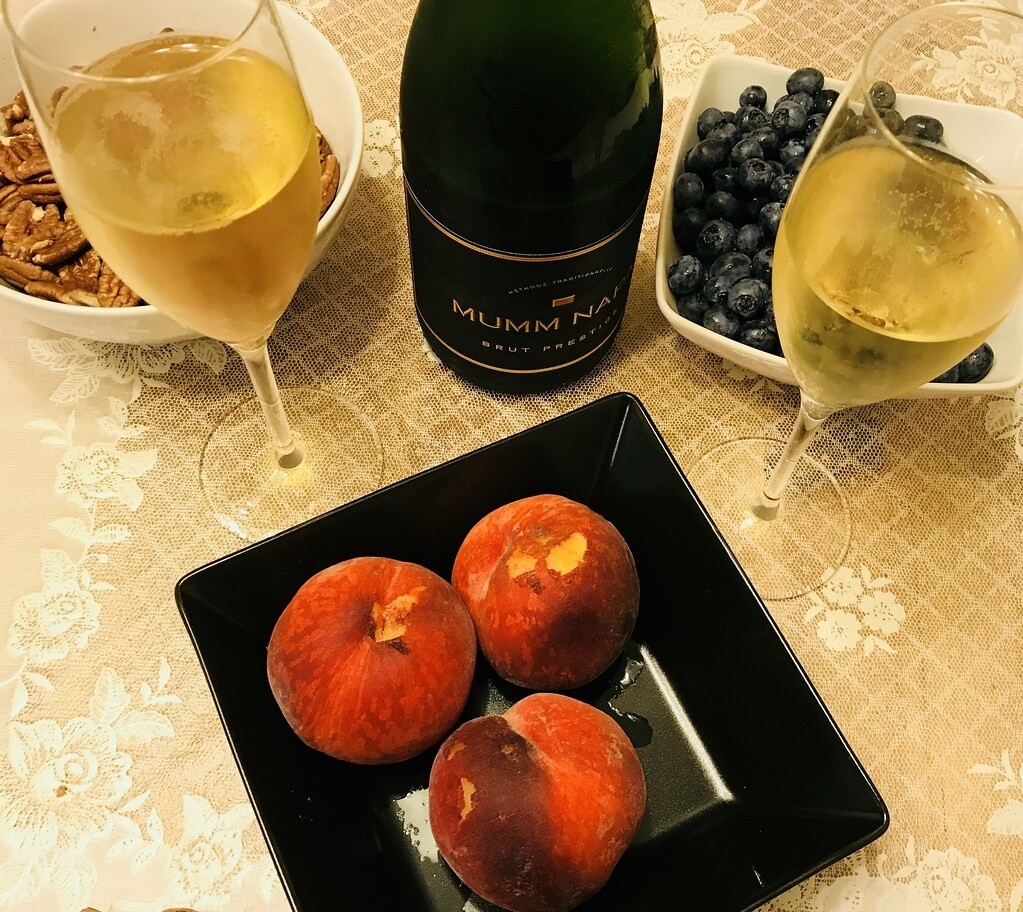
[429,689,646,912]
[451,494,639,691]
[266,556,478,765]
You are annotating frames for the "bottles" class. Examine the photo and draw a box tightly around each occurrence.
[400,0,663,389]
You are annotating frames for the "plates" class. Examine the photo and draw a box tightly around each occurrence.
[173,393,890,912]
[655,56,1023,400]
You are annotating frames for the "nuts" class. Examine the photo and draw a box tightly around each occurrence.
[1,65,340,309]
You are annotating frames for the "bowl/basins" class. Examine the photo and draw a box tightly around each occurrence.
[0,1,364,347]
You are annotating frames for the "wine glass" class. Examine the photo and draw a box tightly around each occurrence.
[1,1,386,546]
[690,2,1023,600]
